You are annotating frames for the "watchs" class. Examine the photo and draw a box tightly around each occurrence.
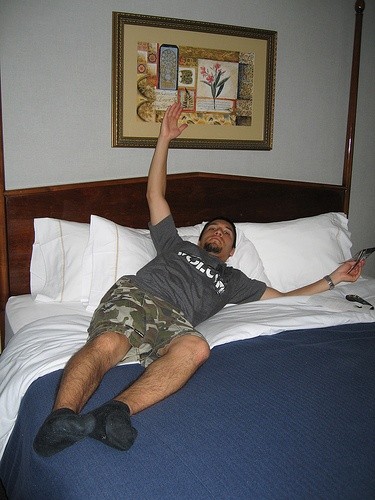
[324,275,334,290]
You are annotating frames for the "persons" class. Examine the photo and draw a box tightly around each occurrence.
[33,103,365,458]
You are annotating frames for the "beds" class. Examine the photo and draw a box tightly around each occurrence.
[0,171,375,499]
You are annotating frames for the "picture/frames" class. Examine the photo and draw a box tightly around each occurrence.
[110,11,278,152]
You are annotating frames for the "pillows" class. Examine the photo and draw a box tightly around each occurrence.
[29,218,200,303]
[200,212,370,294]
[80,214,271,313]
